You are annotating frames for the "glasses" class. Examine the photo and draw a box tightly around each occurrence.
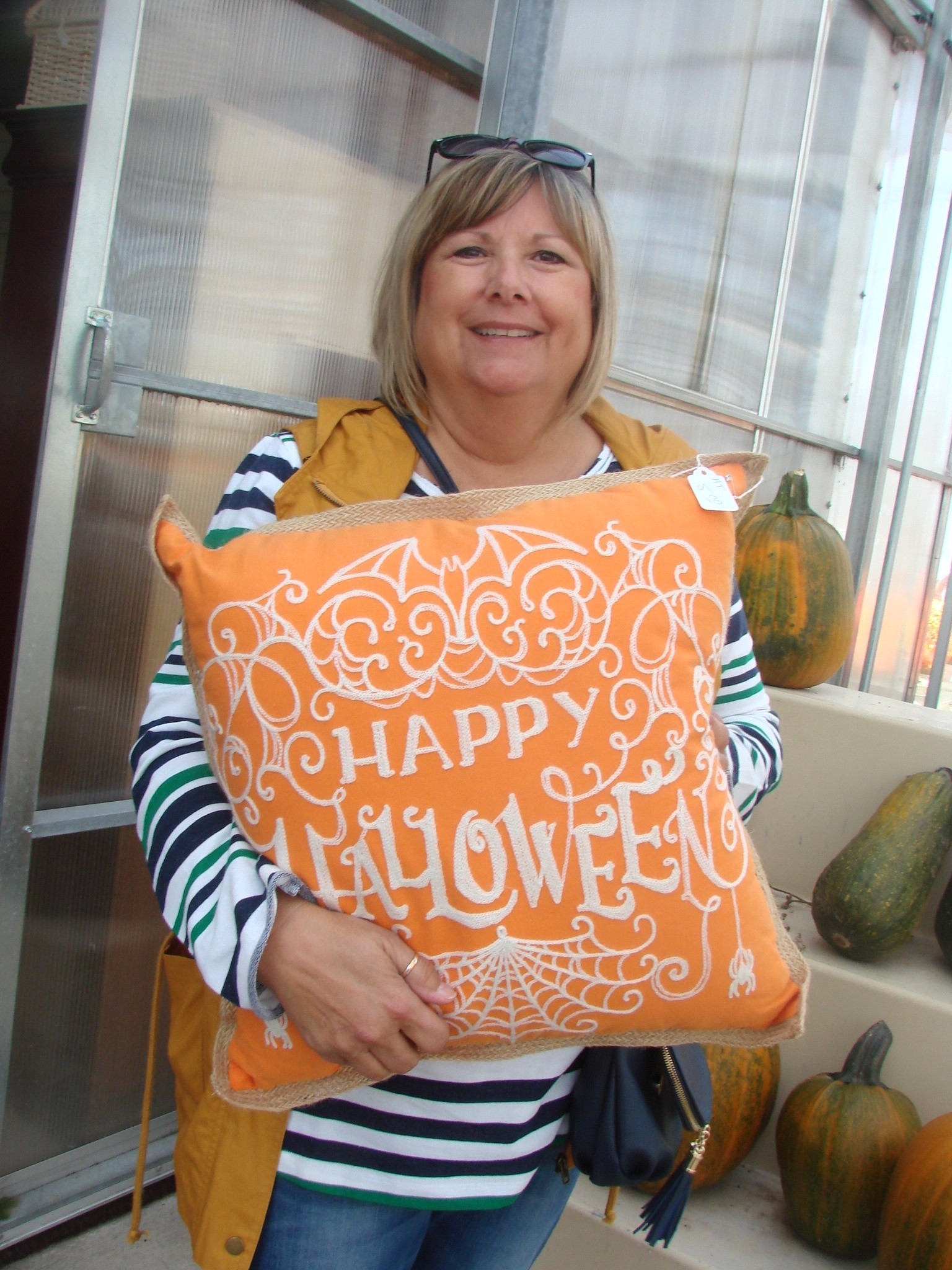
[424,134,597,198]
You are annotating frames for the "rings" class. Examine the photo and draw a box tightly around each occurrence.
[401,953,421,978]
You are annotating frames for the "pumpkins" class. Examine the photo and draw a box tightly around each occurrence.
[774,1018,924,1261]
[810,767,952,963]
[733,467,857,690]
[875,1112,952,1269]
[629,1043,780,1195]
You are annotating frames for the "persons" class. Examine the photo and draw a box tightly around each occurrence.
[125,134,799,1270]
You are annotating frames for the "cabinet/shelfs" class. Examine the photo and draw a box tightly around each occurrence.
[531,679,952,1270]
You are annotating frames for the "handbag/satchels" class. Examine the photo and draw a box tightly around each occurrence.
[566,1037,715,1251]
